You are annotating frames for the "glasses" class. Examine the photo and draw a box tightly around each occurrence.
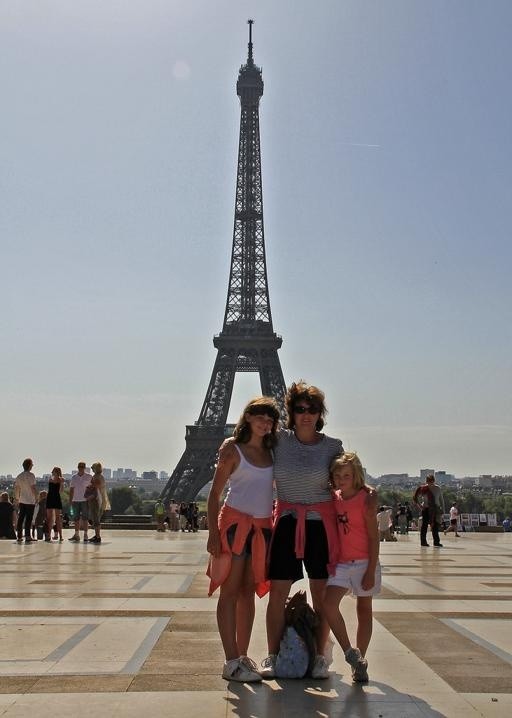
[294,406,321,414]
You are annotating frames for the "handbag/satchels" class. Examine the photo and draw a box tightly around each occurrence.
[84,488,97,497]
[275,590,318,679]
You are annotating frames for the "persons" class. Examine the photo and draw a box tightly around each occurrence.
[216,377,378,679]
[379,501,412,542]
[155,499,208,532]
[323,452,380,681]
[207,397,280,683]
[444,502,460,536]
[503,517,510,531]
[413,473,445,546]
[0,459,112,543]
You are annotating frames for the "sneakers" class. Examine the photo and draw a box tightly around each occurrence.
[84,535,101,542]
[18,537,38,541]
[444,530,446,535]
[311,655,330,679]
[456,535,460,537]
[45,536,64,542]
[222,656,263,683]
[68,535,80,540]
[261,655,277,679]
[422,544,429,546]
[345,647,369,682]
[434,544,443,546]
